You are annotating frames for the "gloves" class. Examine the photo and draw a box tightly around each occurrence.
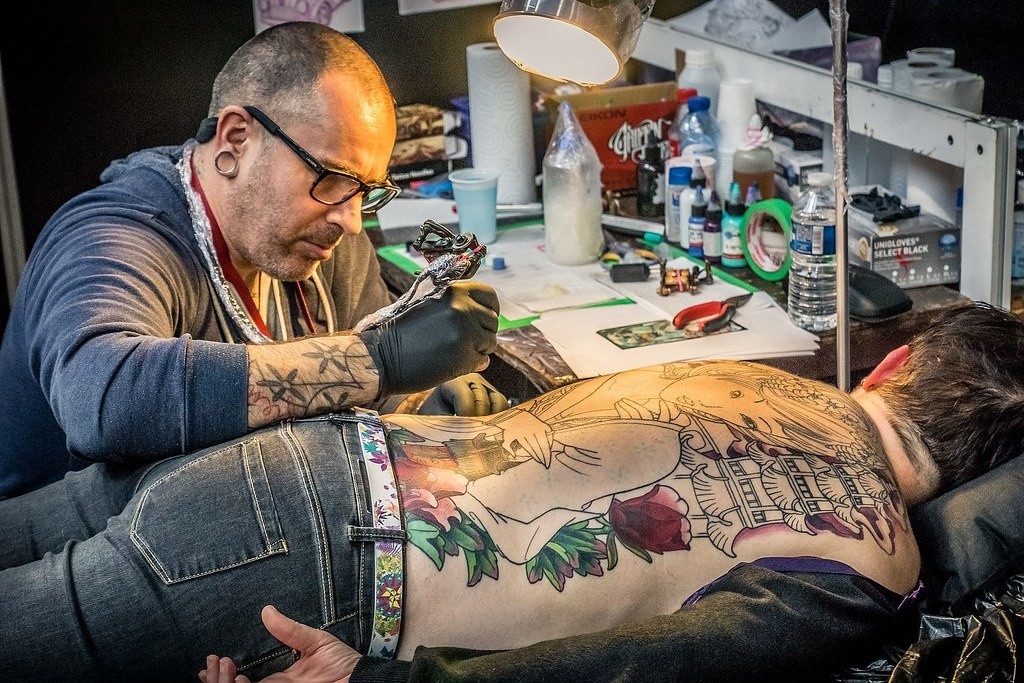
[414,371,512,417]
[352,280,501,401]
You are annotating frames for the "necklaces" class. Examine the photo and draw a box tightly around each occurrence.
[256,270,264,314]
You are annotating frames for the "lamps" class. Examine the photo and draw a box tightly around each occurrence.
[492,1,851,391]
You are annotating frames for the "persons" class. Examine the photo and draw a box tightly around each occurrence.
[0,18,510,497]
[2,299,1024,682]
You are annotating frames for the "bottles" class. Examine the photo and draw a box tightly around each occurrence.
[679,159,712,250]
[689,185,709,259]
[702,190,722,264]
[668,88,698,158]
[542,101,607,266]
[679,96,724,174]
[724,179,762,218]
[787,171,839,331]
[721,183,749,267]
[678,46,722,119]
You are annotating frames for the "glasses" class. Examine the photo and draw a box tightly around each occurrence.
[196,105,403,216]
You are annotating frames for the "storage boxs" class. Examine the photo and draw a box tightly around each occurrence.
[849,184,962,290]
[542,78,679,197]
[387,103,467,190]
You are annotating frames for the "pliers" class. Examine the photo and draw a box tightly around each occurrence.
[672,292,755,332]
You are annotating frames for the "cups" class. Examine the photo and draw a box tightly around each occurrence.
[714,78,757,210]
[448,167,502,246]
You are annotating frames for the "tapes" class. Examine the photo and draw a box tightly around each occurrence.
[742,197,793,283]
